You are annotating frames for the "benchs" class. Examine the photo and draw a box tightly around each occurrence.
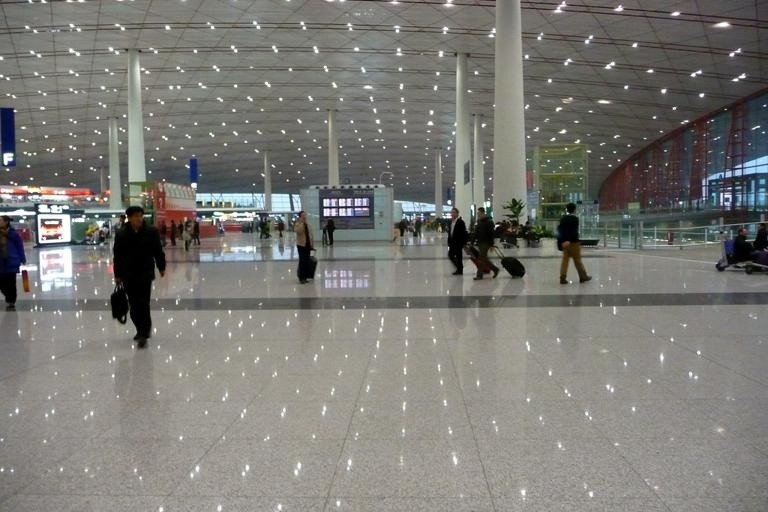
[716,238,768,273]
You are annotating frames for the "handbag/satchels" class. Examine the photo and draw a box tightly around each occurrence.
[110,282,130,325]
[21,269,31,293]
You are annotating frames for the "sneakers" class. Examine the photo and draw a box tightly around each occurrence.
[452,268,463,275]
[300,279,309,284]
[133,330,151,349]
[560,275,592,284]
[473,267,499,280]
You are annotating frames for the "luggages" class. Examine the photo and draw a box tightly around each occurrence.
[493,245,527,279]
[306,248,318,280]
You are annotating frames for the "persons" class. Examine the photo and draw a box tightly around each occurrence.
[733,227,761,262]
[558,203,593,284]
[161,218,201,251]
[294,211,313,283]
[114,214,126,233]
[753,223,768,251]
[448,207,534,279]
[398,217,450,237]
[325,217,334,245]
[0,215,26,309]
[112,205,166,349]
[259,215,286,238]
[448,274,499,343]
